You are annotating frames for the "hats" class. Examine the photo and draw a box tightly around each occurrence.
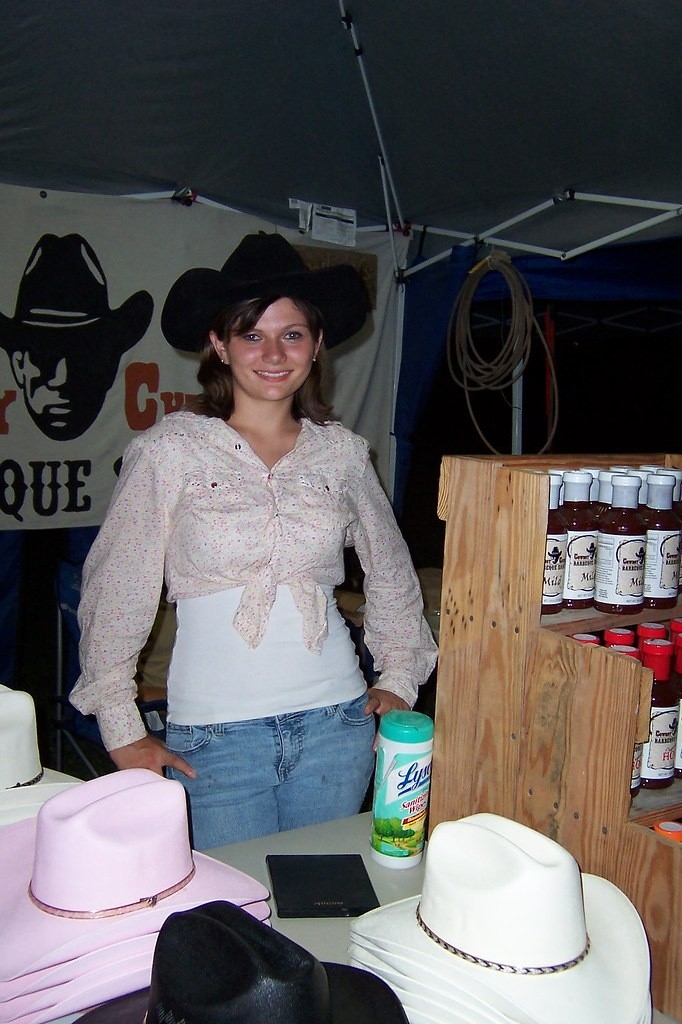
[346,813,652,1024]
[0,768,272,1024]
[162,228,373,358]
[68,900,411,1024]
[0,683,84,808]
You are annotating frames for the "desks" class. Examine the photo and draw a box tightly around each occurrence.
[406,567,443,634]
[195,811,673,1024]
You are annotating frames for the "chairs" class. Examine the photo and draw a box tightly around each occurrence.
[55,595,126,779]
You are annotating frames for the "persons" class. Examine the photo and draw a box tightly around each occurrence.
[70,230,438,852]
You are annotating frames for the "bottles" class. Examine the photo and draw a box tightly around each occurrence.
[370,710,435,870]
[537,463,682,842]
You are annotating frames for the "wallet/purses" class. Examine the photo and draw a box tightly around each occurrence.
[265,854,380,919]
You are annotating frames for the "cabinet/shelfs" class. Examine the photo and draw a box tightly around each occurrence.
[427,454,682,1024]
